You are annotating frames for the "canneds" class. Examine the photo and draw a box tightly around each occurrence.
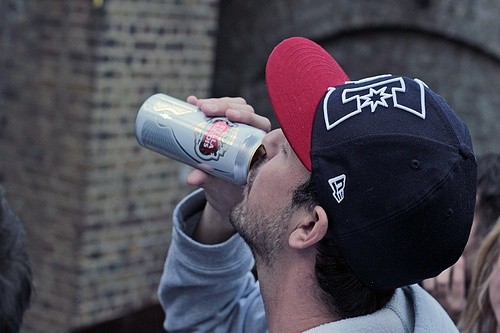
[134,92,269,188]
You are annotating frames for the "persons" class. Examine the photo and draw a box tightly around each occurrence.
[156,36,478,333]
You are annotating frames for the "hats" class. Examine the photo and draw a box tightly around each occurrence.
[267,36,477,291]
